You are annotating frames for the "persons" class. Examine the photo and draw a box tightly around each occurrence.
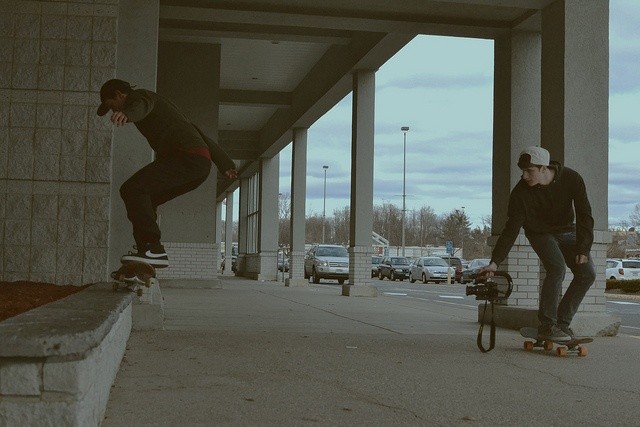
[477,146,597,345]
[96,79,239,268]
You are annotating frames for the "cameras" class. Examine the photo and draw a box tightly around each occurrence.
[466,271,513,301]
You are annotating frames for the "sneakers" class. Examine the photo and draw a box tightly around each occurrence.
[121,245,168,268]
[538,324,571,340]
[111,265,145,285]
[560,327,574,340]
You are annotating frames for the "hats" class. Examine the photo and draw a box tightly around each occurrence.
[517,146,550,167]
[97,79,129,116]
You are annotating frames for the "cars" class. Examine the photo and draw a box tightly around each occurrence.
[381,257,410,281]
[371,257,381,277]
[462,259,491,285]
[278,255,289,272]
[442,257,462,283]
[304,244,350,285]
[410,257,455,284]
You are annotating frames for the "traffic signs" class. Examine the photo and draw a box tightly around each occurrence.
[445,240,454,254]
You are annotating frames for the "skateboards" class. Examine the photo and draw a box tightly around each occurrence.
[520,325,595,357]
[112,261,156,296]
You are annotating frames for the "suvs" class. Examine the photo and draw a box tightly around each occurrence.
[606,258,640,280]
